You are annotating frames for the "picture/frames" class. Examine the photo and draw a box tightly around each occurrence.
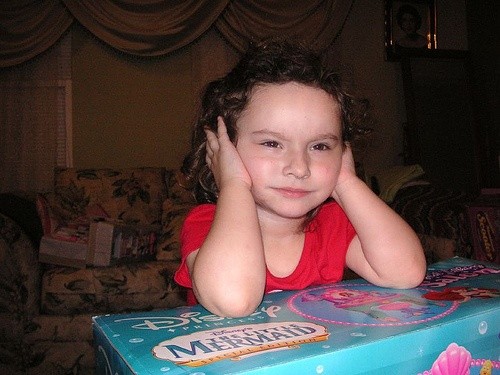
[383,0,438,53]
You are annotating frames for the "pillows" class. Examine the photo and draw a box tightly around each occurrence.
[158,198,195,261]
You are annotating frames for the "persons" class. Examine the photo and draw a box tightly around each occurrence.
[173,36,426,318]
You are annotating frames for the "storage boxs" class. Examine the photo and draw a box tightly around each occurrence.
[83,220,152,267]
[95,256,500,375]
[37,222,89,269]
[468,188,500,264]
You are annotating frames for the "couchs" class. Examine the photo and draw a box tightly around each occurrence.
[0,166,476,375]
